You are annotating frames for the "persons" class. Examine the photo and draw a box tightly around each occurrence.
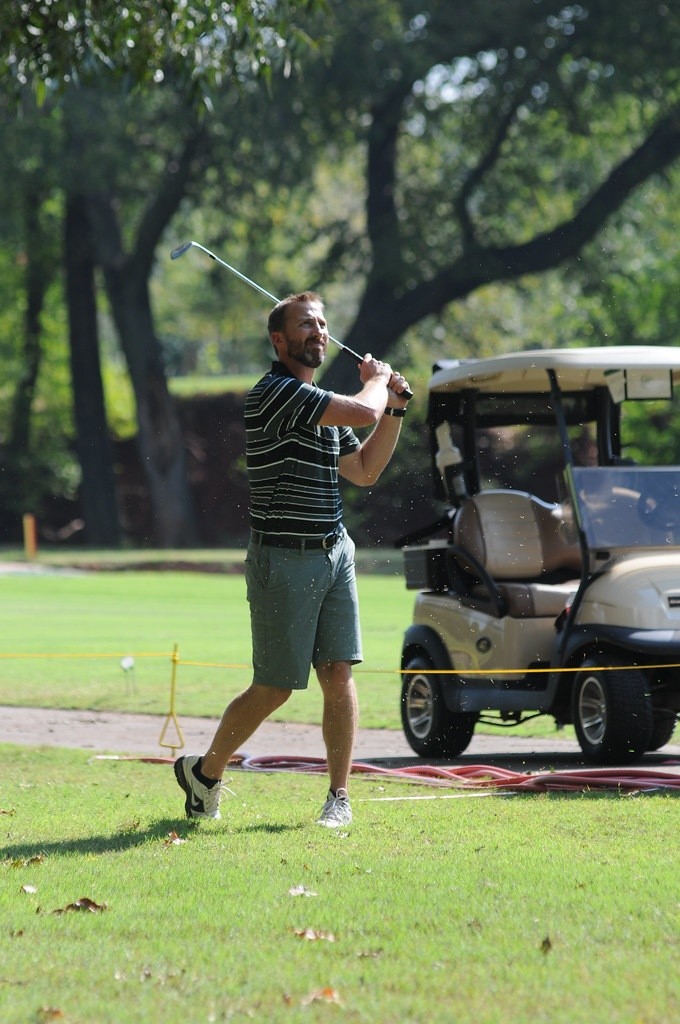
[174,291,410,827]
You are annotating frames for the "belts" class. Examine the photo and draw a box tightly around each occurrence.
[250,522,344,549]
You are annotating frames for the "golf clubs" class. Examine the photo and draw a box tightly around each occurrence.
[170,240,414,401]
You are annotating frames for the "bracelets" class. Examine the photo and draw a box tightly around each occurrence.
[384,407,407,417]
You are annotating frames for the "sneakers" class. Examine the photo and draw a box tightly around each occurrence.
[173,755,222,825]
[315,786,353,831]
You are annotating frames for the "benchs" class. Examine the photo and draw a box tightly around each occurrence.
[451,481,673,618]
